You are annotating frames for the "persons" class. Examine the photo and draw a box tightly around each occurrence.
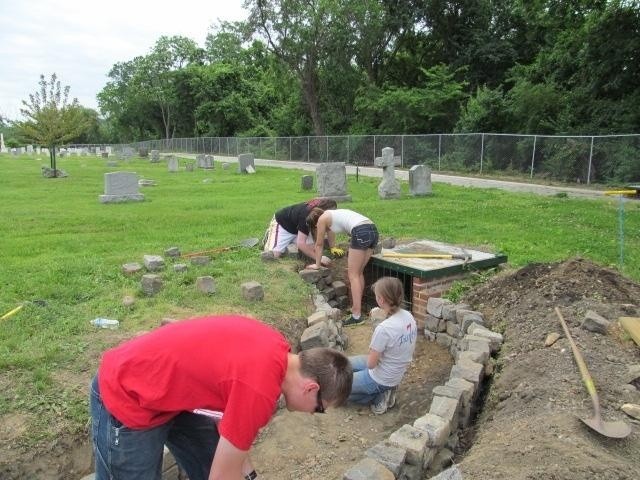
[264,196,338,264]
[305,207,379,327]
[88,316,354,479]
[346,277,420,416]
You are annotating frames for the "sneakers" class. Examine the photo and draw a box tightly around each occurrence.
[342,308,365,326]
[262,229,270,245]
[370,390,395,414]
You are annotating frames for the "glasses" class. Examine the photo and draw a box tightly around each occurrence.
[315,376,325,413]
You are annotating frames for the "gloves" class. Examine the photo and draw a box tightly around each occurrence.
[330,246,345,257]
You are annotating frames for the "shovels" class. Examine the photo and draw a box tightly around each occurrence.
[182,238,260,257]
[554,307,631,438]
